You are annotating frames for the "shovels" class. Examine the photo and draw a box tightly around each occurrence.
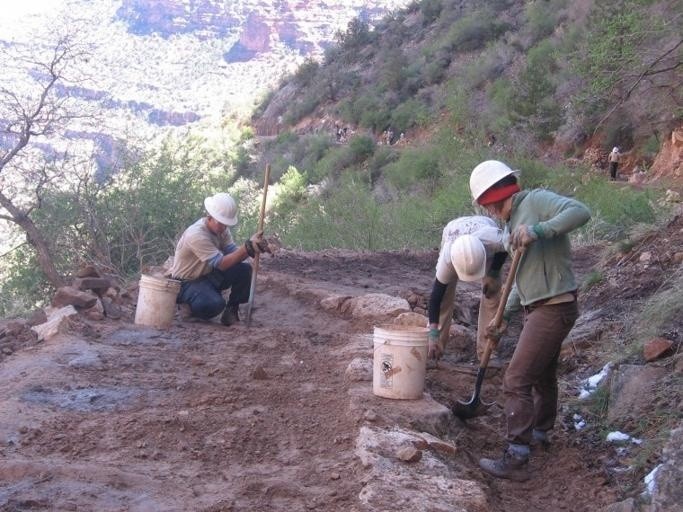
[450,249,522,419]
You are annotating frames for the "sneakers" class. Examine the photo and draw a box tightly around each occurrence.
[486,355,502,369]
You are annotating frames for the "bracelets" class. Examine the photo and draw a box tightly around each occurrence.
[429,326,440,340]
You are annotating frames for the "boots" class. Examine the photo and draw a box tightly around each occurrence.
[179,303,200,323]
[221,301,240,327]
[530,429,547,457]
[479,440,530,482]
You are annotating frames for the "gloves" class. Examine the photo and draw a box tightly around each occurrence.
[245,230,280,257]
[428,328,444,360]
[485,313,508,343]
[508,223,542,253]
[482,270,499,299]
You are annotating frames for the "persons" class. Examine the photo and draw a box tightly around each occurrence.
[423,214,519,376]
[607,146,621,181]
[468,156,593,483]
[169,193,268,329]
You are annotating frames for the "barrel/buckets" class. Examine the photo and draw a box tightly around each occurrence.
[373,325,431,400]
[134,273,183,326]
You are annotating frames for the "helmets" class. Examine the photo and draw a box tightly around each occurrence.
[470,160,521,207]
[450,234,486,282]
[204,192,237,226]
[613,147,619,153]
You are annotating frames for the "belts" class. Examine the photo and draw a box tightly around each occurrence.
[541,292,576,306]
